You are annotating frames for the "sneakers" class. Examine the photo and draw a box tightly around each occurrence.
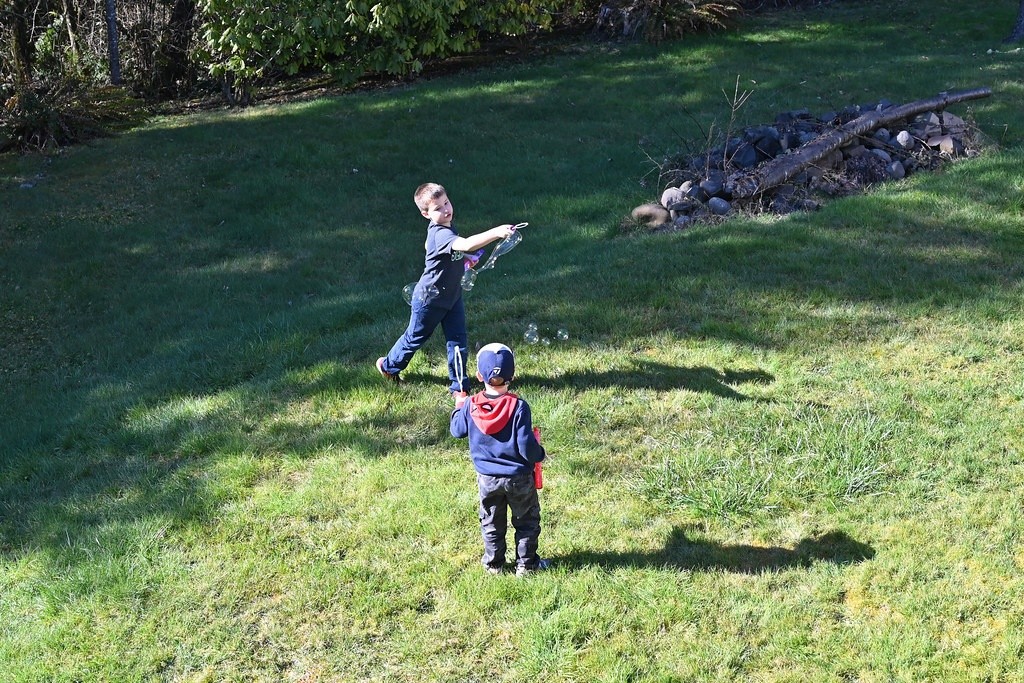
[376,357,407,388]
[450,390,470,407]
[480,553,507,576]
[515,559,550,576]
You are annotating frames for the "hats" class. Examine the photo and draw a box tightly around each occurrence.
[477,343,515,387]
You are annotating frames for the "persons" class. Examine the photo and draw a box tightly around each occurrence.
[376,182,515,403]
[450,342,554,579]
